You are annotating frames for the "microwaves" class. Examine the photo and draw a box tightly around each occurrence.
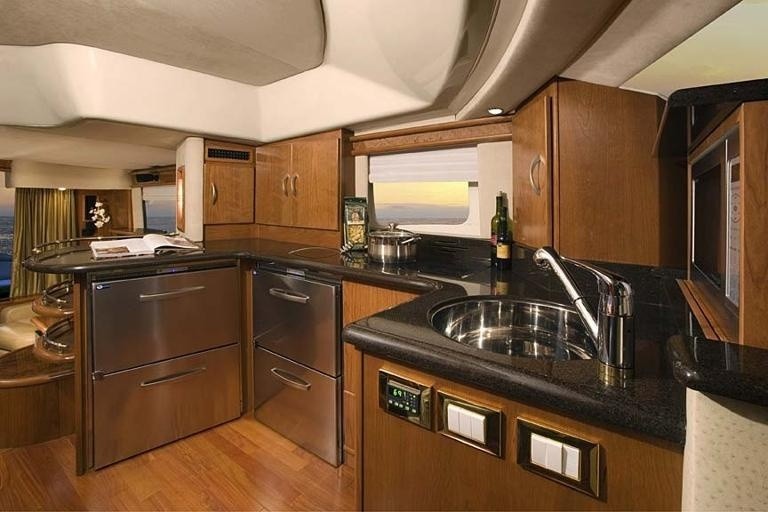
[365,247,493,282]
[685,125,747,307]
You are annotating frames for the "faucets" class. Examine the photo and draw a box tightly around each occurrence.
[531,245,637,388]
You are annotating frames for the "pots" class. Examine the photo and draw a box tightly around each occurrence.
[366,222,422,264]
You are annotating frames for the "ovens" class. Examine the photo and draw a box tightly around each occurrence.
[92,262,244,466]
[252,265,343,469]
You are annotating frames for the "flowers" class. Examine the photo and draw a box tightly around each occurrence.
[89,201,111,229]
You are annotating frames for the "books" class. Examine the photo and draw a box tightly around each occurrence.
[90,233,201,259]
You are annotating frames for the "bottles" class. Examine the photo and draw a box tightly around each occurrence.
[490,195,513,270]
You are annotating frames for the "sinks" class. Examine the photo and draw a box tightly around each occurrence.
[425,291,600,363]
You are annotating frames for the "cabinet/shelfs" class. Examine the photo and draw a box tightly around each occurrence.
[510,72,680,267]
[203,163,255,242]
[337,280,422,471]
[251,129,355,248]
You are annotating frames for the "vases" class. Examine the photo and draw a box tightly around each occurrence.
[97,227,103,239]
